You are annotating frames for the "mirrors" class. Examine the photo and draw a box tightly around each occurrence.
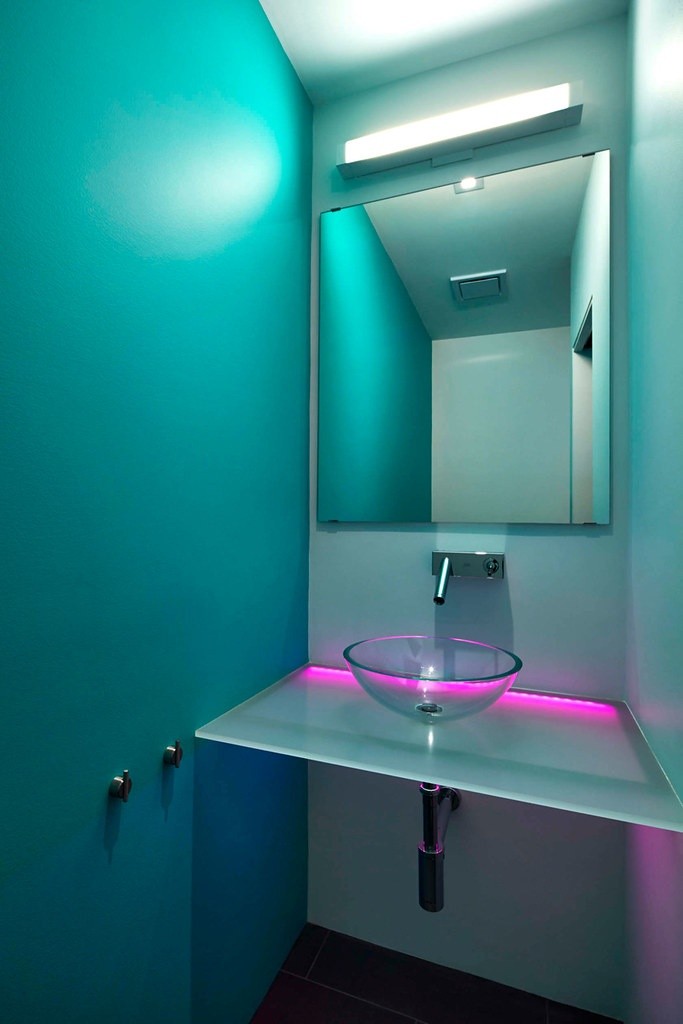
[315,148,613,530]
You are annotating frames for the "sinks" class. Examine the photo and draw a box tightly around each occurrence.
[341,634,523,725]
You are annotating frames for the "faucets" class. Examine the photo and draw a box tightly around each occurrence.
[432,556,452,606]
